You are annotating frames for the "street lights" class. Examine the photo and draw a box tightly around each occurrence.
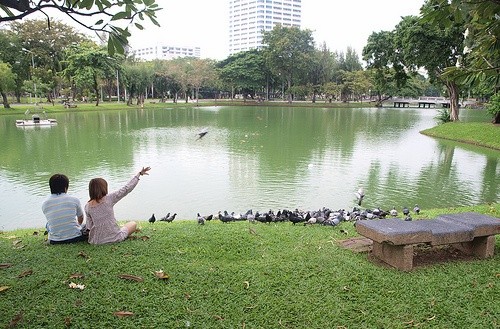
[106,56,120,104]
[22,47,37,104]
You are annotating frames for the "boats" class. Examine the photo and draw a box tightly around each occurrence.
[15,109,57,128]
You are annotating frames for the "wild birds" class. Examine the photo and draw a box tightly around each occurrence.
[198,132,208,138]
[355,188,365,206]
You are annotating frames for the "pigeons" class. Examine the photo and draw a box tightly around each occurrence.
[147,208,422,227]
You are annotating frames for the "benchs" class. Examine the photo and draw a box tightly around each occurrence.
[356,213,500,271]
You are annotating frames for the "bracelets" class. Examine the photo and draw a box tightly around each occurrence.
[139,172,142,175]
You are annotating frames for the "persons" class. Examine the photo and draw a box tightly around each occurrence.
[41,174,88,245]
[85,166,151,245]
